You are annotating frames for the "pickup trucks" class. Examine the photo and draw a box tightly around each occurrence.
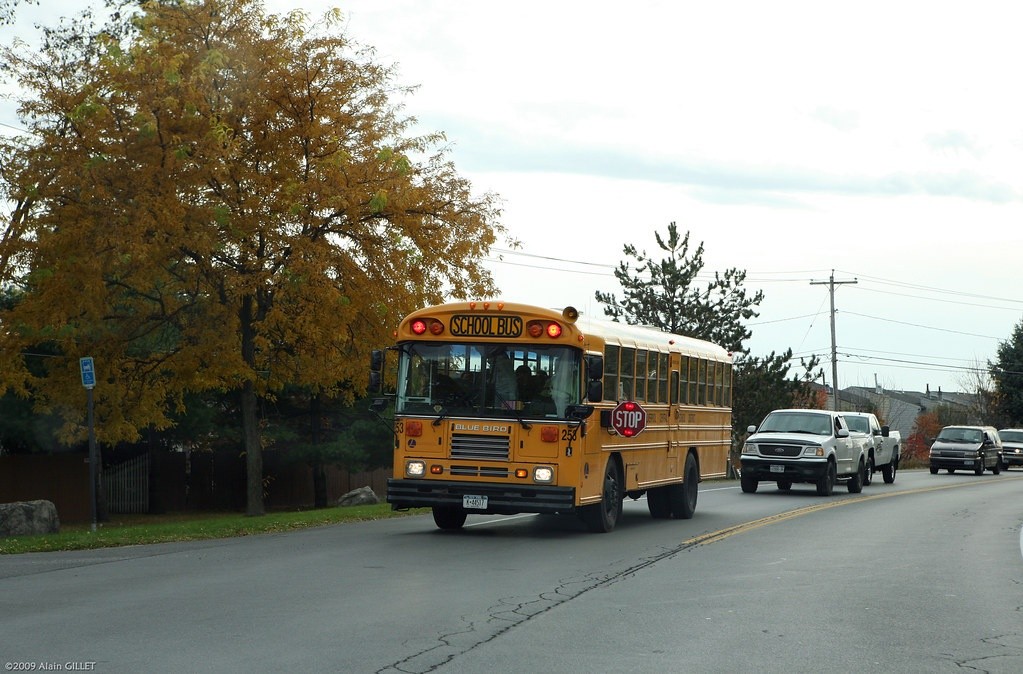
[832,411,906,486]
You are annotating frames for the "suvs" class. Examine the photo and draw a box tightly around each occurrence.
[928,425,1003,477]
[996,428,1023,471]
[739,408,853,497]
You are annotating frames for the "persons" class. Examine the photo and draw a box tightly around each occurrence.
[417,359,549,410]
[817,417,839,434]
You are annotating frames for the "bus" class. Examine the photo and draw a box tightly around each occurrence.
[368,301,734,532]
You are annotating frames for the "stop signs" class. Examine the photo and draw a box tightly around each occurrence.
[611,401,646,438]
